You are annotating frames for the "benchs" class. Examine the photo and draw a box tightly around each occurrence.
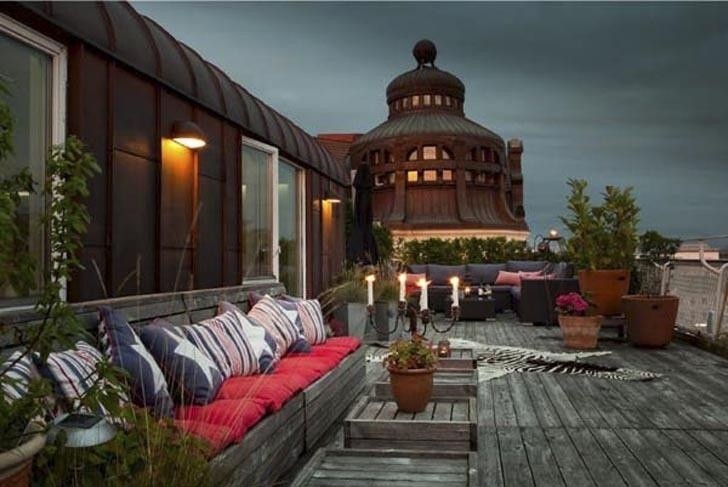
[183,279,369,449]
[0,282,306,487]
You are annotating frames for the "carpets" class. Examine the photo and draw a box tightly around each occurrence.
[448,335,668,382]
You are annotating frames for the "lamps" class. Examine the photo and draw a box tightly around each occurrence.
[170,119,206,151]
[324,191,342,204]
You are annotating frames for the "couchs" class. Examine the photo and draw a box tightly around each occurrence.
[406,260,578,325]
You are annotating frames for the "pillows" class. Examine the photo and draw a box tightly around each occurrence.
[410,261,576,285]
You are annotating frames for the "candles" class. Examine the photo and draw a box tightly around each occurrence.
[417,279,430,309]
[398,272,407,303]
[365,274,376,306]
[449,276,460,305]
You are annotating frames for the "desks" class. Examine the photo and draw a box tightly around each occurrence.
[443,294,496,322]
[290,348,480,487]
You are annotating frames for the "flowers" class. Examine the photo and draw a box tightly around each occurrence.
[552,289,599,316]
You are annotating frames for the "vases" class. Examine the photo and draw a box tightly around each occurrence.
[557,315,604,350]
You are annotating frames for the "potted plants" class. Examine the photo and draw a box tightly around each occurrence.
[333,259,368,341]
[381,334,437,412]
[557,177,643,317]
[0,67,104,486]
[372,275,398,341]
[621,230,684,348]
